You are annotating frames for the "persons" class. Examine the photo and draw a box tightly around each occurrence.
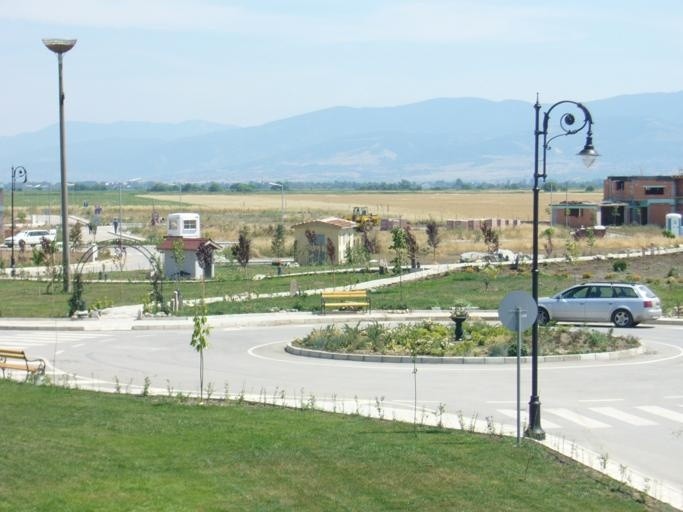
[113,218,119,234]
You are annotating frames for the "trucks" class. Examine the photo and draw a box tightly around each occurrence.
[568,225,606,241]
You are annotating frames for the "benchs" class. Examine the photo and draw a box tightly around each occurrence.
[0,348,46,383]
[320,289,372,316]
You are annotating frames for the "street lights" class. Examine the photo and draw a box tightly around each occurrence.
[117,182,129,251]
[0,166,35,278]
[42,37,77,293]
[270,182,284,227]
[169,183,182,212]
[523,91,601,442]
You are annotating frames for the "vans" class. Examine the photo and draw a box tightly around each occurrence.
[537,283,663,328]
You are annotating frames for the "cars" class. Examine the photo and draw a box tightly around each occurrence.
[5,230,57,248]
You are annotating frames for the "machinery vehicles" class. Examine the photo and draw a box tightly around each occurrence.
[342,206,382,231]
